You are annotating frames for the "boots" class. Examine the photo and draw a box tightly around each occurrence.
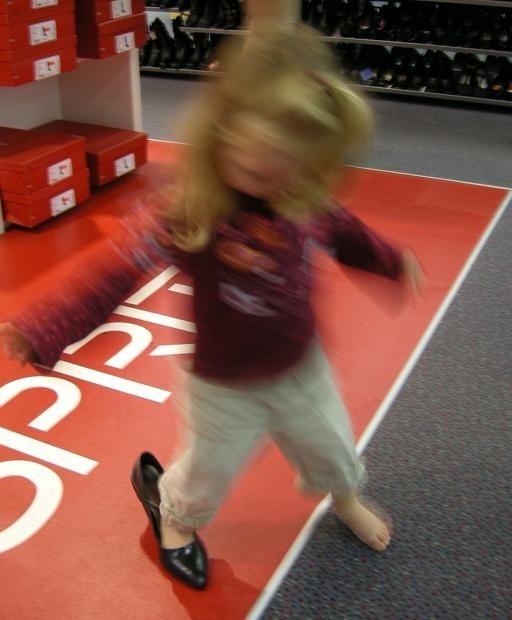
[139,2,244,70]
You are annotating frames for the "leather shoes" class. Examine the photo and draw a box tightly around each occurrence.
[329,41,512,101]
[301,0,512,52]
[130,447,209,593]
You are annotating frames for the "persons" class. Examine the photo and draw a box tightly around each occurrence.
[1,21,431,553]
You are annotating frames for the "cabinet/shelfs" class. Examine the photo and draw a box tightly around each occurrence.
[143,21,511,109]
